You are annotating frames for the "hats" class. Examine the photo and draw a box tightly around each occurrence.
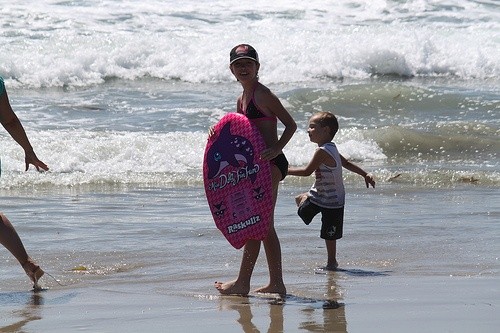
[229,44,260,66]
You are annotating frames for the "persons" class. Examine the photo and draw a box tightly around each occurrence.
[287,112,376,270]
[206,44,298,293]
[0,77,49,286]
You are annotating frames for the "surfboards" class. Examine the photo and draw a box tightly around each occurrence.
[201,112,276,250]
